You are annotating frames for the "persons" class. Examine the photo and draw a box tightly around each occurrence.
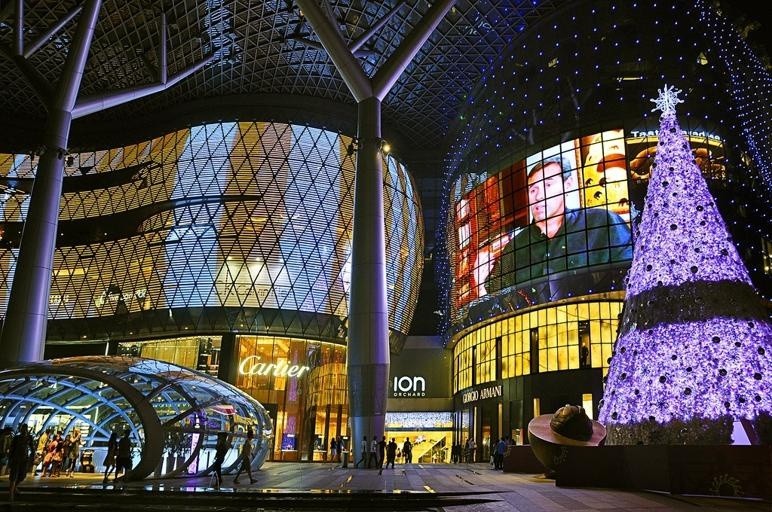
[484,433,517,470]
[1,420,82,504]
[336,436,345,462]
[485,156,635,293]
[386,436,413,469]
[368,436,379,468]
[355,436,369,468]
[330,438,338,463]
[102,429,135,483]
[214,432,230,489]
[375,436,386,469]
[233,430,259,485]
[451,437,475,464]
[416,435,446,464]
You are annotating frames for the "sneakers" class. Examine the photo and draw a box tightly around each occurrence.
[233,478,257,484]
